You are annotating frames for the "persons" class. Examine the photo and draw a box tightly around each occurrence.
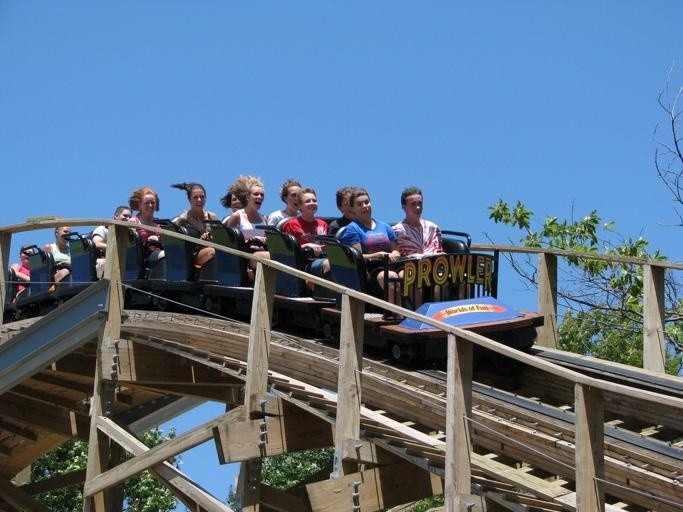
[92,205,133,279]
[44,226,72,292]
[174,183,220,266]
[330,186,401,319]
[268,179,331,289]
[12,243,33,298]
[128,188,166,259]
[394,187,446,306]
[221,174,270,279]
